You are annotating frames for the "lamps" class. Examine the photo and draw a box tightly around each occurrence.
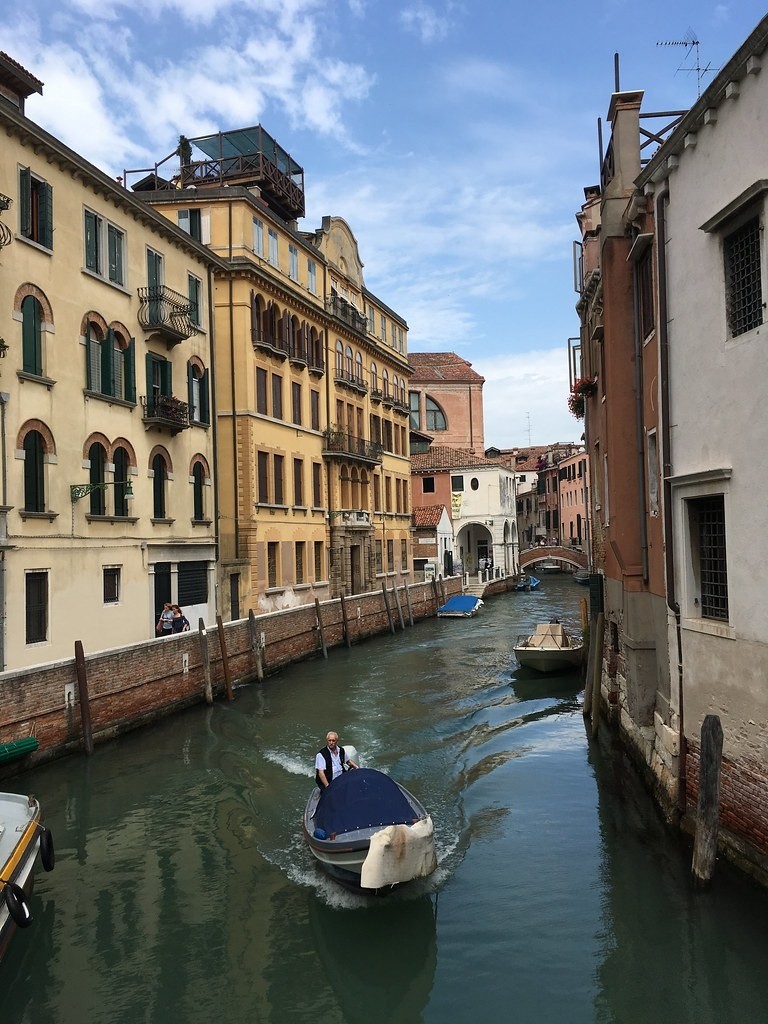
[69,479,135,503]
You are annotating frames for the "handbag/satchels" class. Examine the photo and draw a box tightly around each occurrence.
[156,612,165,632]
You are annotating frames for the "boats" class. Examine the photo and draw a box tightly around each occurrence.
[513,617,585,671]
[0,792,55,964]
[516,575,540,591]
[302,745,438,897]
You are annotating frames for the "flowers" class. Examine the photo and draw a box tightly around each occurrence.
[567,394,585,421]
[572,375,601,398]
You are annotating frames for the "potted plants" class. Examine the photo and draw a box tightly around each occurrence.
[321,421,353,451]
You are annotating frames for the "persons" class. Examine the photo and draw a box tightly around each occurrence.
[551,537,557,545]
[172,605,189,634]
[540,537,547,546]
[535,541,538,547]
[159,603,174,636]
[314,732,359,790]
[550,615,561,623]
[530,543,533,549]
[179,135,192,164]
